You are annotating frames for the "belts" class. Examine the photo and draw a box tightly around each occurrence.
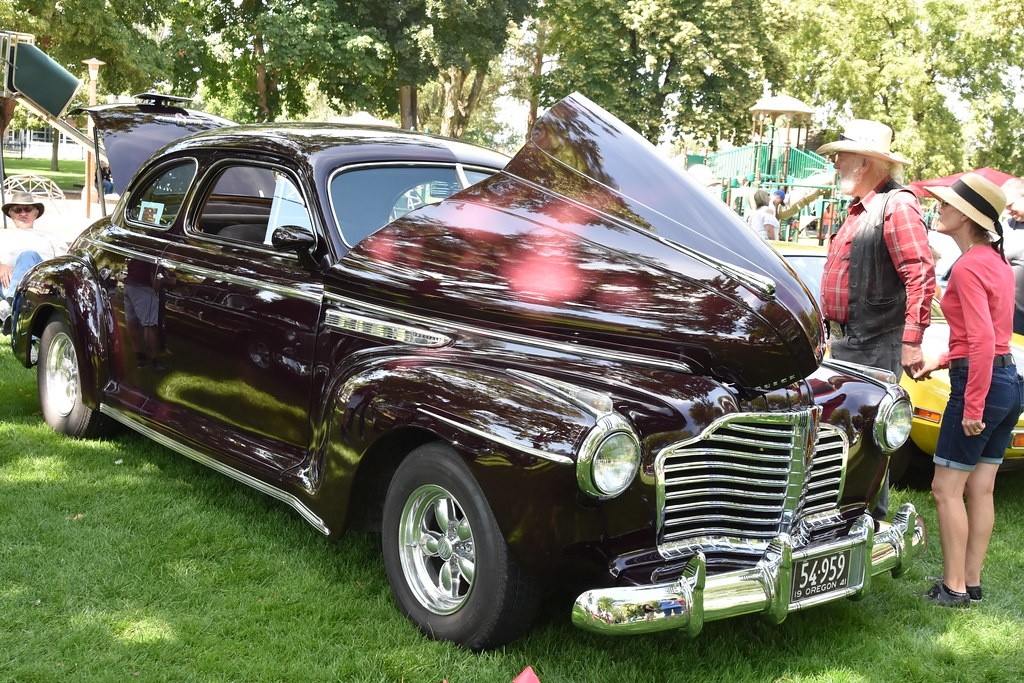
[826,319,857,340]
[948,354,1015,369]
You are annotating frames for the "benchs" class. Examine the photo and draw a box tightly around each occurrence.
[160,214,269,234]
[74,183,106,192]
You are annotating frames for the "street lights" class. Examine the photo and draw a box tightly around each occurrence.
[80,59,109,205]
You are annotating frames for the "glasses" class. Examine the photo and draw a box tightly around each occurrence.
[10,207,35,213]
[839,133,854,141]
[939,199,950,209]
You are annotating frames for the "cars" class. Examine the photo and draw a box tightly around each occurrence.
[0,88,926,657]
[760,236,1024,489]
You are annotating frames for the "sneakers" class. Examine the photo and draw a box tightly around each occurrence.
[967,586,983,602]
[0,300,12,321]
[922,584,971,609]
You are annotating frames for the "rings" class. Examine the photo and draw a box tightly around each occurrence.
[976,428,980,433]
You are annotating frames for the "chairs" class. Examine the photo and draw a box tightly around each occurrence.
[217,224,268,243]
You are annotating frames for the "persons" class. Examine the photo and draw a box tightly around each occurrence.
[737,118,1024,611]
[0,191,55,336]
[94,159,113,205]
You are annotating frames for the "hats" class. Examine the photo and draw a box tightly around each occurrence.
[1,192,44,219]
[774,190,786,207]
[923,172,1007,234]
[816,119,912,165]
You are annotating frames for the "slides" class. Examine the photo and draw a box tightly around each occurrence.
[688,163,722,198]
[775,170,833,220]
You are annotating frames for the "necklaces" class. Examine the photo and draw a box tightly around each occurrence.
[961,238,987,256]
